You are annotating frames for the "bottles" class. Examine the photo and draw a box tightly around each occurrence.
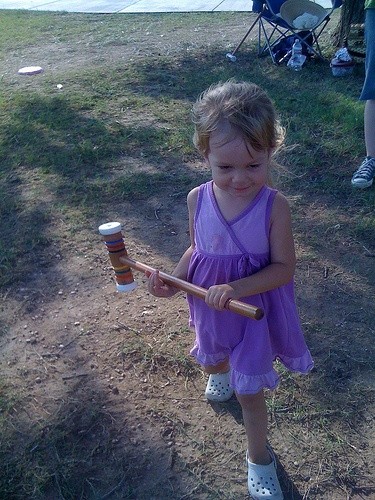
[226,53,236,62]
[292,39,302,72]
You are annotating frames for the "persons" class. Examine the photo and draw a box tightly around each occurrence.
[145,80,315,500]
[351,0,375,189]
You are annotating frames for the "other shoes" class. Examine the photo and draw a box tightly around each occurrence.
[351,155,375,188]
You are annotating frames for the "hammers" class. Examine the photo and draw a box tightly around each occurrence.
[98,222,264,320]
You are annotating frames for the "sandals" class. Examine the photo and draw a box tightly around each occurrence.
[246,443,285,500]
[204,370,233,402]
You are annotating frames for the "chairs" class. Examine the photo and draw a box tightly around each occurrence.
[252,0,335,67]
